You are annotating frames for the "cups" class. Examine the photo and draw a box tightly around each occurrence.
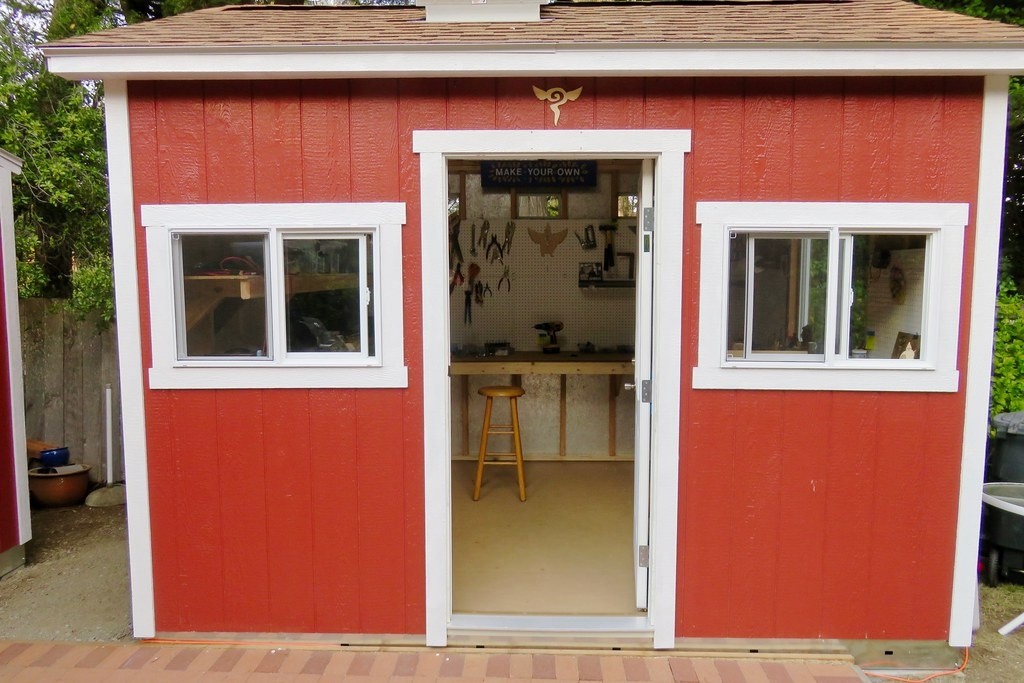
[808,341,818,354]
[852,349,868,358]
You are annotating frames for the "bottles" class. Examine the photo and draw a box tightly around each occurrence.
[866,330,876,351]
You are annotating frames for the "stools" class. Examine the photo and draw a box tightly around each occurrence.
[472,385,527,503]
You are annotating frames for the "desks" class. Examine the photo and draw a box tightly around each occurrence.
[448,351,634,462]
